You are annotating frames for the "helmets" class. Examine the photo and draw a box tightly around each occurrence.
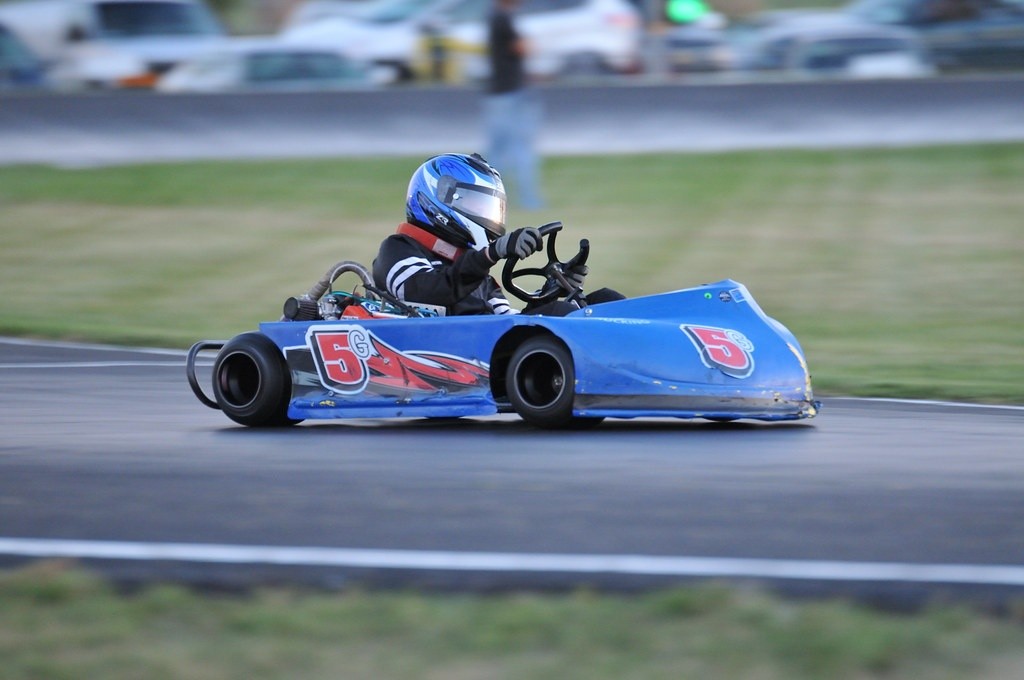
[405,153,505,250]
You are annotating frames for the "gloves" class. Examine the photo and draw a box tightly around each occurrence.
[488,226,542,264]
[541,267,588,296]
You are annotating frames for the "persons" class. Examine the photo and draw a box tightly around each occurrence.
[470,0,548,211]
[372,152,628,317]
